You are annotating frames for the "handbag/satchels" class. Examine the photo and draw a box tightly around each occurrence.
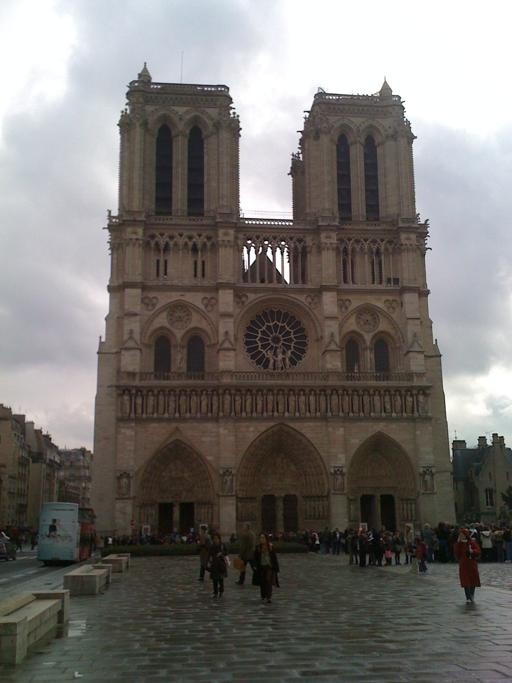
[233,559,245,571]
[221,543,231,569]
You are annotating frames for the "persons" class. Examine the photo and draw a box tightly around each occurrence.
[198,523,281,602]
[268,528,346,555]
[453,529,481,601]
[346,523,512,573]
[103,532,201,548]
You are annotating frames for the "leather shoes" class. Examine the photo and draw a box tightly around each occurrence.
[198,577,273,603]
[466,595,475,602]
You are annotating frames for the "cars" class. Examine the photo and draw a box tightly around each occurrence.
[0,542,18,561]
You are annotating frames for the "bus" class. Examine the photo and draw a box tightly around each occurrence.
[36,501,98,565]
